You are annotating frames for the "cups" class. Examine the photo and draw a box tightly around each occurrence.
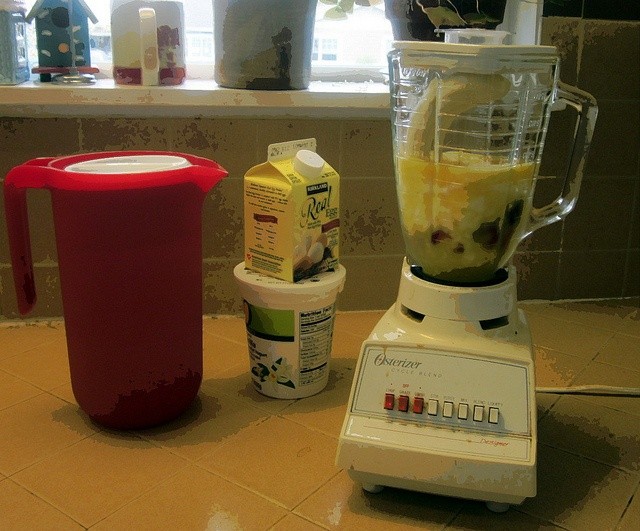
[2,150,230,431]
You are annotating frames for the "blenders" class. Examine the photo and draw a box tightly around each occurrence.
[331,26,600,514]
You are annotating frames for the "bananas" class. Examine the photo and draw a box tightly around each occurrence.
[404,75,512,161]
